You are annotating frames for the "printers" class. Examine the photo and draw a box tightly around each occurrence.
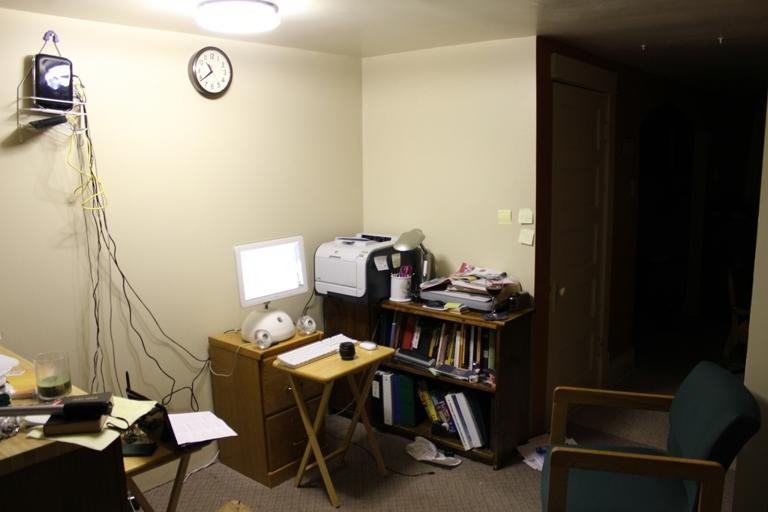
[314,232,436,306]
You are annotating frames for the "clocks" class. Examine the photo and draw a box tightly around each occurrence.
[189,46,234,99]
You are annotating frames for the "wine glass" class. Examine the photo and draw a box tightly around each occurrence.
[485,274,503,314]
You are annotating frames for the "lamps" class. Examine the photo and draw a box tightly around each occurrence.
[194,0,283,35]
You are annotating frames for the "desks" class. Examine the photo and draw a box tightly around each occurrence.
[273,340,397,508]
[1,343,129,512]
[117,425,215,511]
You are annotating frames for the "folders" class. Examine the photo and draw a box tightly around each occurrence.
[125,370,175,443]
[370,370,425,428]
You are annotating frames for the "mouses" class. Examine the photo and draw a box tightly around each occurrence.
[360,340,377,351]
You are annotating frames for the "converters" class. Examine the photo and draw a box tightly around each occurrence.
[33,53,73,109]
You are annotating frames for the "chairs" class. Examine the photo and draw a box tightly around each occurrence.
[541,360,763,511]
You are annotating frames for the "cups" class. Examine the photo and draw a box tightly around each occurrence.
[389,273,412,302]
[35,351,71,401]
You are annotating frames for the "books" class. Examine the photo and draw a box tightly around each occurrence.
[371,314,496,452]
[44,390,116,437]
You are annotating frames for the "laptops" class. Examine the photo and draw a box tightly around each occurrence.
[125,388,213,454]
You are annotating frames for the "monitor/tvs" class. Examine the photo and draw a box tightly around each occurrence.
[232,235,309,345]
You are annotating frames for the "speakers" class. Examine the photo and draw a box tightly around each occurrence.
[296,314,316,335]
[253,329,272,349]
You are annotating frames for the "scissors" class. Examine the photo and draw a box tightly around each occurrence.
[400,265,413,277]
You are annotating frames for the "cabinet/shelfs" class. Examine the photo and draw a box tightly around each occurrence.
[208,327,327,489]
[372,299,534,471]
[322,296,381,422]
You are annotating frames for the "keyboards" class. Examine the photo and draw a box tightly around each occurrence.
[277,333,358,370]
